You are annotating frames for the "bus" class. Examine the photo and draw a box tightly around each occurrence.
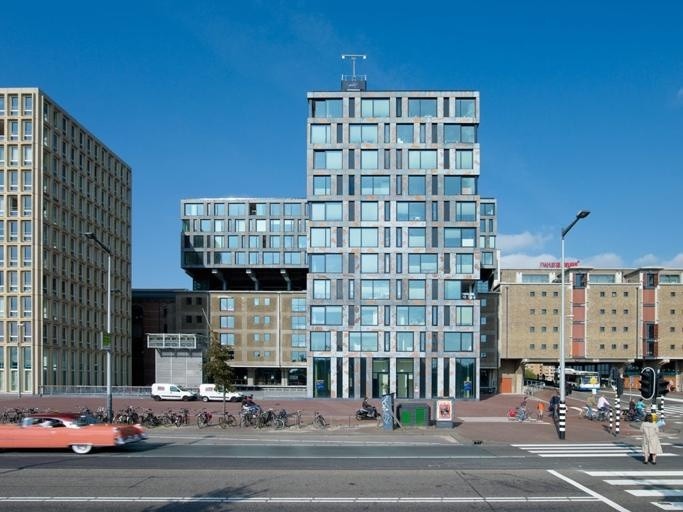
[553,368,601,392]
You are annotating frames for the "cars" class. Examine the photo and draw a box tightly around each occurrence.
[0,413,145,454]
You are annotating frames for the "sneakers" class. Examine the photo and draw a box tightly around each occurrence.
[644,460,656,465]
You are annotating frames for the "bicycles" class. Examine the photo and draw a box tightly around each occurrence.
[220,405,326,428]
[2,408,36,422]
[506,407,539,423]
[82,408,188,426]
[579,406,648,423]
[196,408,212,428]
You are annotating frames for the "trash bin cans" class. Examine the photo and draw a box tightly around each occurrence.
[396,403,431,426]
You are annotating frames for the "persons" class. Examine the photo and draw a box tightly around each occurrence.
[518,397,528,424]
[598,392,612,415]
[640,414,664,465]
[241,394,255,410]
[565,380,572,396]
[548,391,561,419]
[627,397,647,421]
[586,394,597,420]
[536,399,544,419]
[362,397,374,417]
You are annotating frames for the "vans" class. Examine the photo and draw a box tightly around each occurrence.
[200,384,243,401]
[151,383,197,402]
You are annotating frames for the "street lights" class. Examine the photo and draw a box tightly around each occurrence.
[84,231,112,422]
[560,210,590,440]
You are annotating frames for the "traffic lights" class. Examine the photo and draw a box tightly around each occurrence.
[656,372,669,398]
[638,367,656,399]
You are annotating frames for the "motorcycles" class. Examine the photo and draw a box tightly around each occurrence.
[356,407,382,421]
